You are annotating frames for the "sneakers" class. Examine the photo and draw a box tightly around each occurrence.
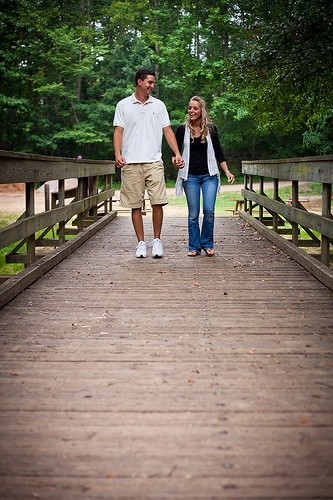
[148,238,163,258]
[135,240,147,258]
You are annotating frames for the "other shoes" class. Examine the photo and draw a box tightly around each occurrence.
[203,248,214,255]
[188,251,199,256]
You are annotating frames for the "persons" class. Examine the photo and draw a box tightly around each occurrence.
[113,70,185,258]
[171,95,235,257]
